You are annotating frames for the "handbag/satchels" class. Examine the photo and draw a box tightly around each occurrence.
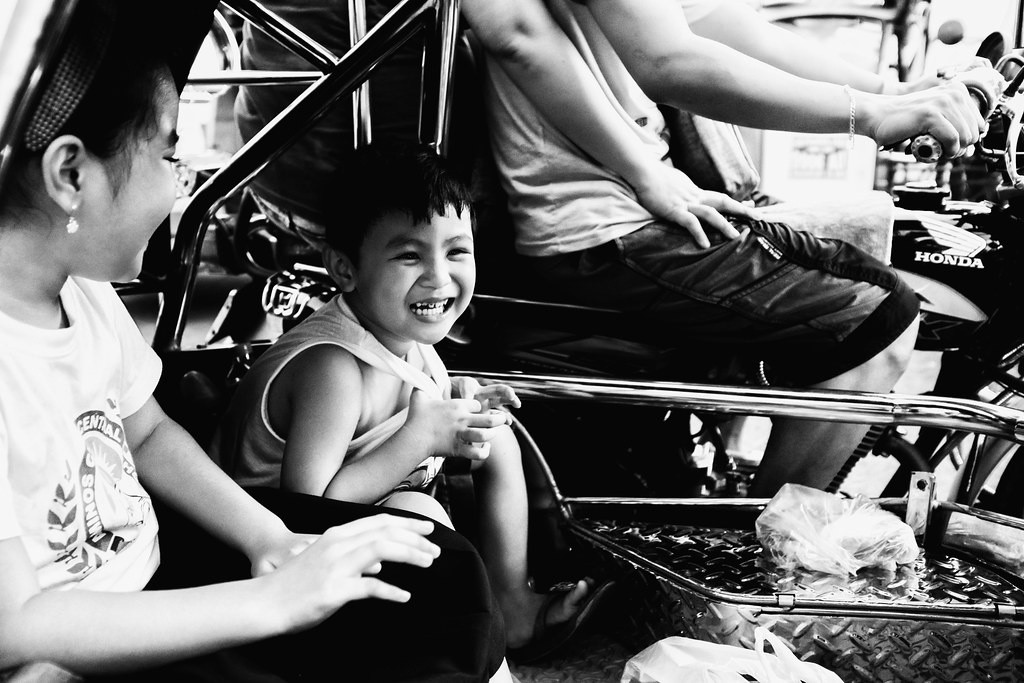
[659,98,759,200]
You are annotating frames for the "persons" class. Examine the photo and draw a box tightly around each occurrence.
[233,142,616,656]
[459,0,989,499]
[0,0,493,683]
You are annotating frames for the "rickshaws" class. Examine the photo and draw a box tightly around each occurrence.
[0,1,1024,683]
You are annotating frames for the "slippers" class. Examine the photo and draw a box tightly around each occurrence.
[504,577,615,662]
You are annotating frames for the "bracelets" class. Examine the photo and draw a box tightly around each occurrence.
[844,84,856,153]
